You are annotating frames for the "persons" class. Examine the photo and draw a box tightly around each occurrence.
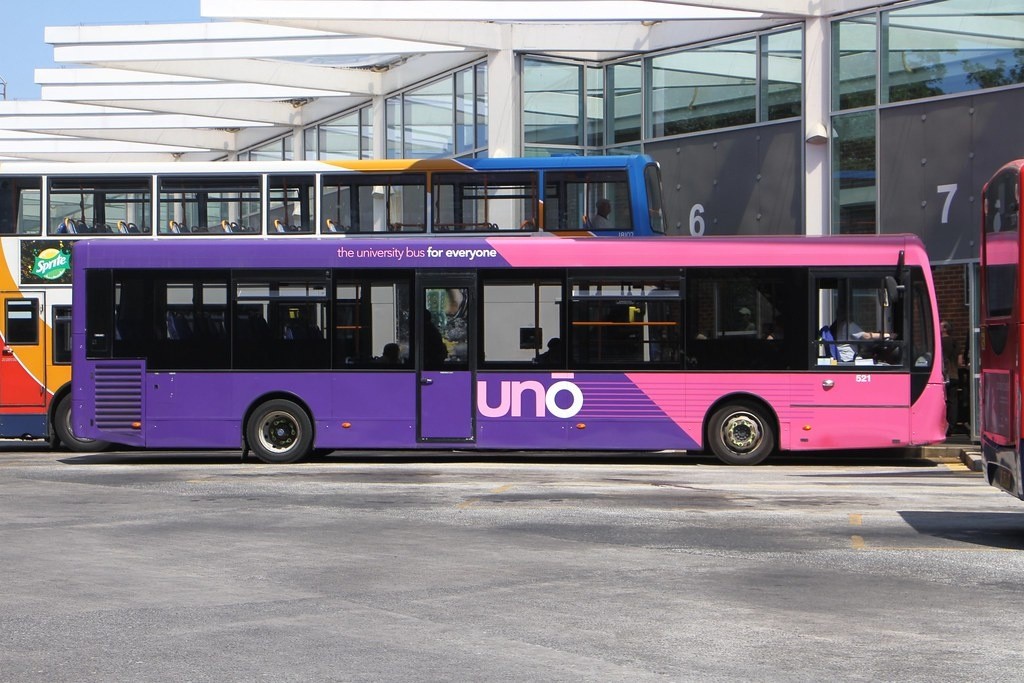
[590,199,616,229]
[937,320,964,437]
[365,309,455,371]
[650,327,679,363]
[764,314,785,341]
[829,303,897,362]
[535,338,561,369]
[733,307,756,339]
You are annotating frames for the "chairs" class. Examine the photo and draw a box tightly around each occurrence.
[820,325,843,362]
[168,313,194,341]
[56,219,346,233]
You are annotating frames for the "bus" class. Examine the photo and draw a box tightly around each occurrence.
[979,157,1024,501]
[70,234,948,465]
[0,153,668,453]
